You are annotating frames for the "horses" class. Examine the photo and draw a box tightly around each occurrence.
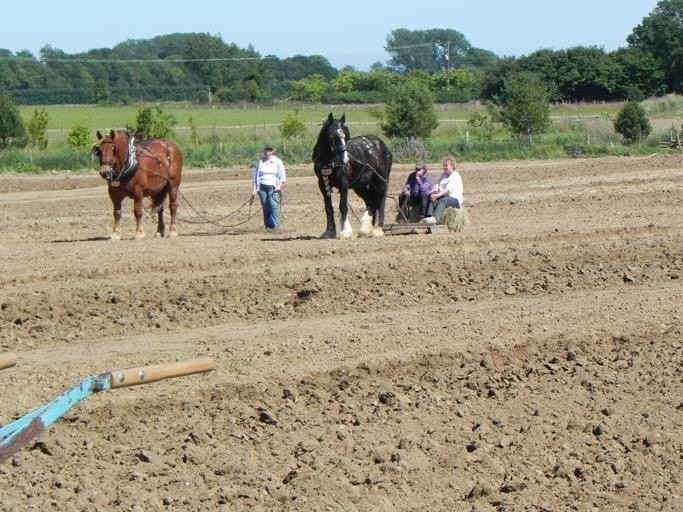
[311,112,392,240]
[95,129,183,242]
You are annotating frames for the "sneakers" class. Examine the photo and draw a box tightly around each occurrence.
[419,218,425,223]
[423,217,436,223]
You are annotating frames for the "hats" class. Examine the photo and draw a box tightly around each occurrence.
[264,144,274,149]
[415,160,425,169]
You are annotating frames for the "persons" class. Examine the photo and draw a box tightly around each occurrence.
[423,157,463,225]
[397,160,431,224]
[252,144,286,230]
